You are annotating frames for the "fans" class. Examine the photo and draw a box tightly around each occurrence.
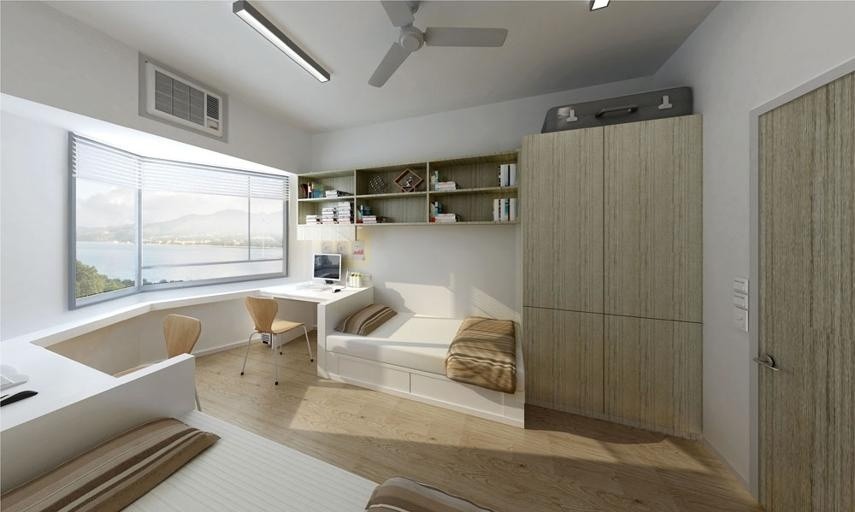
[368,0,508,88]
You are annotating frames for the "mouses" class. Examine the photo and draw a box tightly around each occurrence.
[332,289,341,293]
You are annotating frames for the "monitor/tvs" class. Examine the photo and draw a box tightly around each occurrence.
[312,253,342,286]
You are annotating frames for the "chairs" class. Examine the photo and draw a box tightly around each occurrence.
[112,313,201,411]
[240,295,313,386]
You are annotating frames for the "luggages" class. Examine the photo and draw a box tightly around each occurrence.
[540,85,696,134]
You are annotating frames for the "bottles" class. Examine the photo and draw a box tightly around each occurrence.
[345,267,362,288]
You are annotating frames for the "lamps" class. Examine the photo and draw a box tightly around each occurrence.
[589,0,610,11]
[232,1,330,83]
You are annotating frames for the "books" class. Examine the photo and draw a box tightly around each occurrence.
[429,171,457,191]
[430,201,457,223]
[499,164,517,188]
[359,205,378,223]
[301,182,352,224]
[493,198,516,222]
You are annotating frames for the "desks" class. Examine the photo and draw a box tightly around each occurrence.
[0,342,119,430]
[261,277,364,348]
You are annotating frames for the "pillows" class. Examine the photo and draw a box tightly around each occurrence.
[1,418,221,511]
[334,303,397,336]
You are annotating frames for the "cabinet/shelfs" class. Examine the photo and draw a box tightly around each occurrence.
[520,114,701,437]
[296,152,520,224]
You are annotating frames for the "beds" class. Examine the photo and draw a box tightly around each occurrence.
[0,354,380,511]
[317,286,525,429]
